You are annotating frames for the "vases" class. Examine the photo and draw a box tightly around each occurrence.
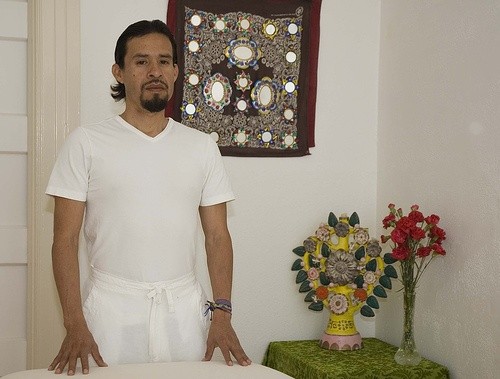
[394,293,421,365]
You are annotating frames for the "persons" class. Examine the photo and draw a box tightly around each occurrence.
[45,20,252,376]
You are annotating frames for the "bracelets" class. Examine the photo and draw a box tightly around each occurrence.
[204,299,232,321]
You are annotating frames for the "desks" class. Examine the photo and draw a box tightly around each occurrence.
[261,338,449,379]
[0,360,294,379]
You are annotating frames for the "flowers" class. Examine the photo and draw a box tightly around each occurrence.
[381,204,447,351]
